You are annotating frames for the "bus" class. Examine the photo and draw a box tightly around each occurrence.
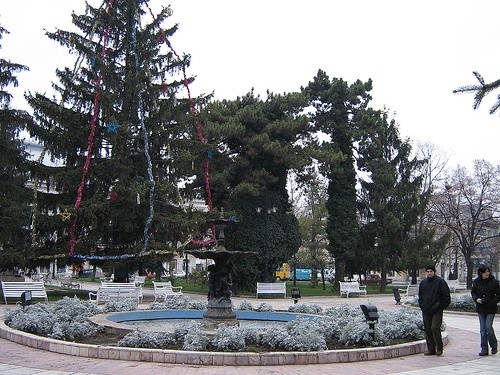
[274,263,290,281]
[291,262,321,281]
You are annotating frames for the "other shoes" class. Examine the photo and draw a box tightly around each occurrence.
[436,350,443,356]
[479,351,489,356]
[424,349,436,355]
[491,340,498,354]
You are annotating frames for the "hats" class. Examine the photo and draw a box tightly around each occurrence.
[426,264,435,271]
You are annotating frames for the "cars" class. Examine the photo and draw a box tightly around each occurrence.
[167,270,189,278]
[58,269,73,277]
[358,274,392,286]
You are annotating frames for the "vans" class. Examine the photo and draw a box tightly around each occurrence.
[81,269,101,279]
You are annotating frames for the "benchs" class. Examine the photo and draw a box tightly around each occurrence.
[152,281,183,303]
[392,277,412,284]
[400,285,418,302]
[1,281,48,304]
[256,282,286,299]
[89,274,147,304]
[445,279,460,294]
[338,281,367,299]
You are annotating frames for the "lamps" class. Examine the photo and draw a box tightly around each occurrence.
[360,304,379,347]
[20,291,33,309]
[290,288,302,304]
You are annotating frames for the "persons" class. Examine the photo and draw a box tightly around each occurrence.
[471,264,500,356]
[419,265,451,356]
